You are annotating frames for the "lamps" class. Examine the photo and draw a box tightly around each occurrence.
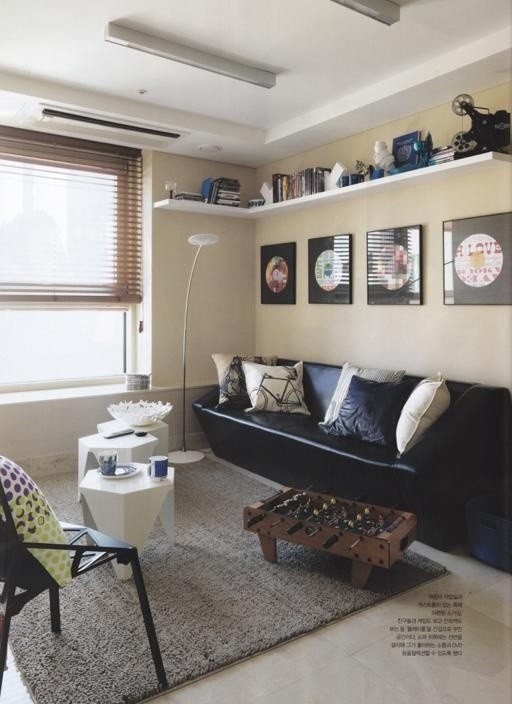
[331,0,401,26]
[167,234,219,464]
[103,23,276,90]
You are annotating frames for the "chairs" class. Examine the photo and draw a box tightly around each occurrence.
[0,482,167,691]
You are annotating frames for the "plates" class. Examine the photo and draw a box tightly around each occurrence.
[96,463,139,480]
[136,430,147,436]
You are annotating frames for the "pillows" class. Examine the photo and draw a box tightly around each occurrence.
[0,456,73,589]
[212,353,452,458]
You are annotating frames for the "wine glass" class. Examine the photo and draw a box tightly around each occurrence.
[165,178,177,199]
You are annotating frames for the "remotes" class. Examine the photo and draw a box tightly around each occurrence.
[104,429,134,438]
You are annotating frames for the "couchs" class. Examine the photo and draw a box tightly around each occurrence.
[192,356,512,553]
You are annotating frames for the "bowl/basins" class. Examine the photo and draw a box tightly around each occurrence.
[248,197,264,209]
[123,373,151,391]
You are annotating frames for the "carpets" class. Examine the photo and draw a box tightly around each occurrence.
[0,457,451,704]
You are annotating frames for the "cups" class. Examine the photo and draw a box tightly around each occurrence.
[147,454,169,478]
[339,175,349,187]
[98,450,118,475]
[349,173,363,184]
